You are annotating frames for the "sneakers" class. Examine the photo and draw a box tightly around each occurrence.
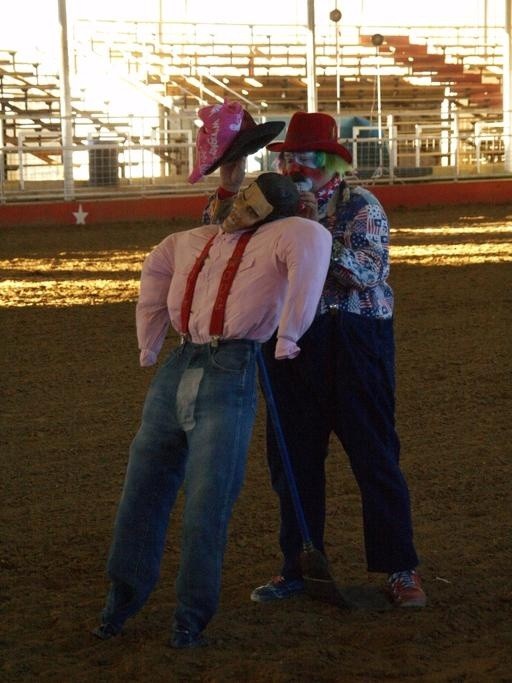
[251,574,307,602]
[92,624,112,639]
[385,571,426,608]
[171,627,196,648]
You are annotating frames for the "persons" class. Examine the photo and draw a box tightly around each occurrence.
[92,168,334,647]
[200,108,429,611]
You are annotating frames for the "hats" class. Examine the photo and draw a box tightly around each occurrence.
[266,111,352,162]
[204,105,285,175]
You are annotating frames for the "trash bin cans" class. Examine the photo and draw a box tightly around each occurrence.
[88,136,119,185]
